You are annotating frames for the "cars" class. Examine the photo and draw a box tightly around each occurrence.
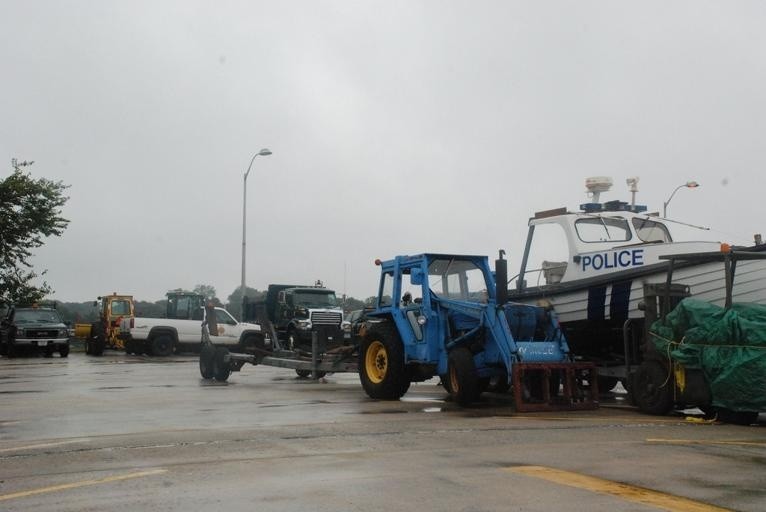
[340,309,364,335]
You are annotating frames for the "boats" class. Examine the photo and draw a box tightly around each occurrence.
[473,172,766,348]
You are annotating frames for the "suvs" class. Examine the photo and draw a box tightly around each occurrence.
[1,302,72,359]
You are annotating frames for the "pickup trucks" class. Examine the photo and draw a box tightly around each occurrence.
[116,301,268,360]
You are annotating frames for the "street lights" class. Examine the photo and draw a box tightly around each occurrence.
[238,145,274,320]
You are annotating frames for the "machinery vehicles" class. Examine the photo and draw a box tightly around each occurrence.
[356,244,601,414]
[81,290,139,357]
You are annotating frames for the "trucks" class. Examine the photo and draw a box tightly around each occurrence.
[237,279,348,349]
